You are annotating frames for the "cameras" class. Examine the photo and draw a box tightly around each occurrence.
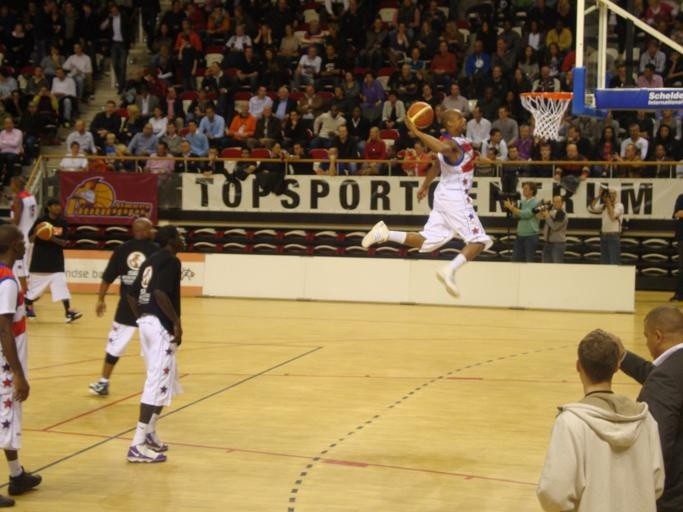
[531,201,553,214]
[603,191,609,198]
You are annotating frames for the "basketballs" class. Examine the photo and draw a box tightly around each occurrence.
[35,222,54,241]
[406,102,433,129]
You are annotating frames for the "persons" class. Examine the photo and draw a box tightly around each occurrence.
[536,307,682,511]
[1,0,682,323]
[1,223,43,506]
[88,217,184,464]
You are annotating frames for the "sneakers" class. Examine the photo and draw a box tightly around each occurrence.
[88,381,108,395]
[127,430,168,462]
[361,220,389,248]
[436,265,460,298]
[1,466,41,507]
[65,310,82,323]
[25,307,36,318]
